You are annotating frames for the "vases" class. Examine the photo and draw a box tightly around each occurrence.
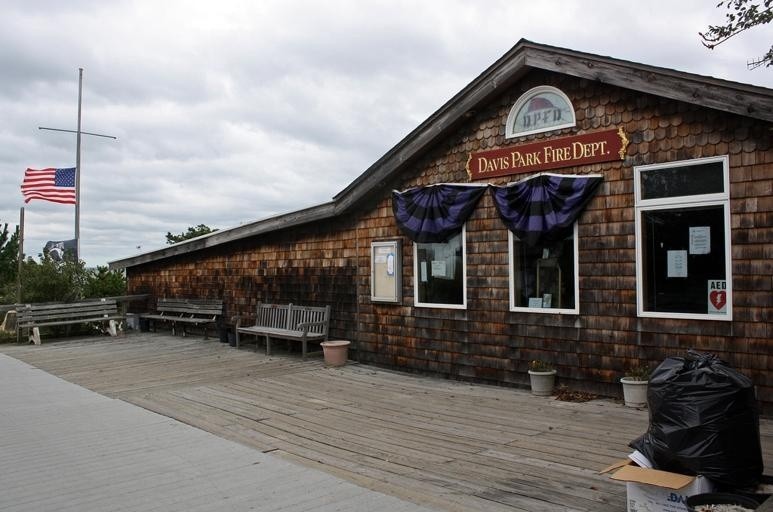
[321,339,351,367]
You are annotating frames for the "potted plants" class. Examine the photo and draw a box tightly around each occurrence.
[526,360,557,396]
[620,364,649,409]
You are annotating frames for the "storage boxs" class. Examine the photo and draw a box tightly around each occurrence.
[598,454,712,511]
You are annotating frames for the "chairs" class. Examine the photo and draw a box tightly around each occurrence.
[236,302,330,357]
[141,298,225,337]
[16,300,124,345]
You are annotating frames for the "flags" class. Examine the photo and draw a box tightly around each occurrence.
[20,166,76,208]
